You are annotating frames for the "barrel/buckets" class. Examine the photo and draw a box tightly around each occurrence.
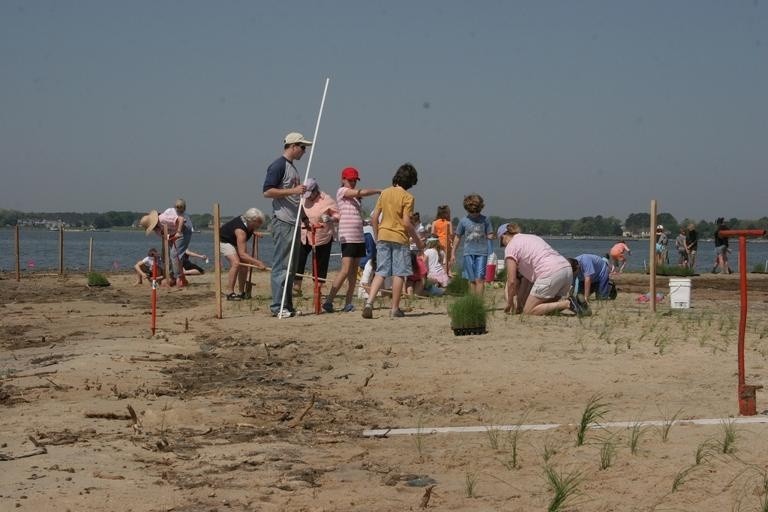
[669,278,693,311]
[496,260,505,278]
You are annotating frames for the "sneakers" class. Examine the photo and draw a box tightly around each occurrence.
[322,303,334,312]
[576,293,592,316]
[227,292,241,300]
[344,304,353,311]
[237,293,245,297]
[390,308,404,317]
[295,310,302,316]
[568,296,583,314]
[609,282,617,299]
[276,308,294,317]
[292,288,302,296]
[362,304,372,317]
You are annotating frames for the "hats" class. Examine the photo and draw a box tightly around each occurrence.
[341,167,361,181]
[302,178,317,198]
[283,133,312,146]
[497,223,509,248]
[139,209,158,237]
[426,238,439,244]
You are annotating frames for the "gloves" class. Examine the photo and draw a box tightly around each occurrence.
[321,215,331,223]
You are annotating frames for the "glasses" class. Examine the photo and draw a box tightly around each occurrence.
[469,210,481,213]
[295,144,305,150]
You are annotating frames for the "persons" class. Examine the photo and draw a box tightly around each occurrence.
[362,163,425,317]
[675,227,689,266]
[610,240,630,274]
[263,132,313,316]
[504,268,533,307]
[714,217,729,273]
[566,254,617,306]
[686,224,697,268]
[402,205,454,299]
[322,167,385,312]
[358,243,390,303]
[291,178,340,296]
[602,253,610,264]
[497,222,593,316]
[451,194,495,296]
[711,247,731,274]
[134,198,207,287]
[219,207,265,301]
[655,224,667,266]
